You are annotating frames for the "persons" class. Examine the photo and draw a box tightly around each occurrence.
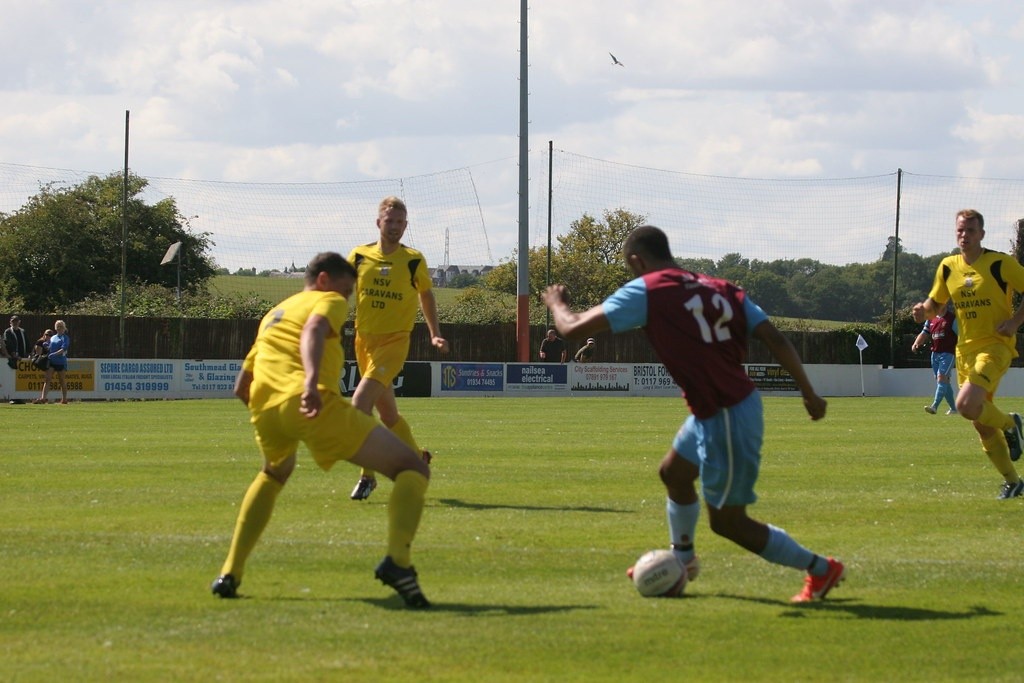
[212,251,433,608]
[34,321,68,403]
[0,316,30,360]
[913,303,958,414]
[543,228,844,598]
[913,209,1024,498]
[28,329,54,358]
[346,198,448,500]
[540,330,566,362]
[575,338,596,362]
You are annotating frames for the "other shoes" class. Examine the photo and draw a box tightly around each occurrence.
[945,410,957,415]
[924,406,937,414]
[34,399,46,404]
[61,399,68,404]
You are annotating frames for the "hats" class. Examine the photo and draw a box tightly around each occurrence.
[587,337,596,344]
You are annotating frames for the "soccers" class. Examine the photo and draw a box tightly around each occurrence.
[632,549,687,598]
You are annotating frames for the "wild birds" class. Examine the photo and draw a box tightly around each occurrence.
[609,52,626,69]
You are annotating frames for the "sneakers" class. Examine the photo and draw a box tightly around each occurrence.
[423,451,432,466]
[374,556,430,608]
[351,477,376,500]
[998,479,1024,499]
[626,557,699,582]
[790,557,845,602]
[1003,412,1024,461]
[213,574,235,598]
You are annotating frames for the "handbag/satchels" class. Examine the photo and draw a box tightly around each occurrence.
[31,355,50,371]
